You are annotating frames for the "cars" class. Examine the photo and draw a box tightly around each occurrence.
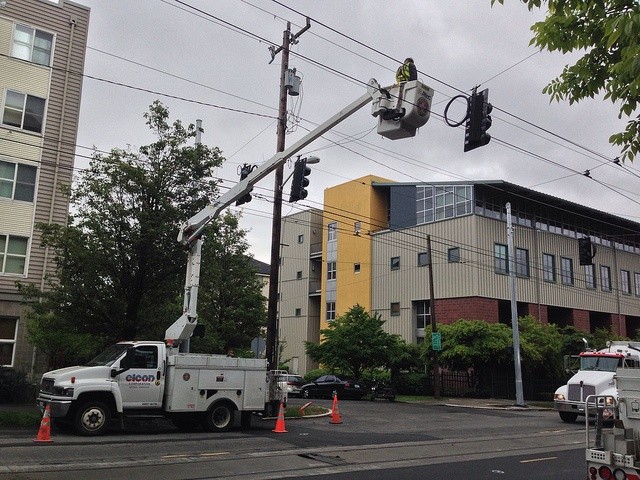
[300,375,367,400]
[274,375,307,398]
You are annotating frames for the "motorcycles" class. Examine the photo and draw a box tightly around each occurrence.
[370,378,396,402]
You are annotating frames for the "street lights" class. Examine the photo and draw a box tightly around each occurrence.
[266,158,319,371]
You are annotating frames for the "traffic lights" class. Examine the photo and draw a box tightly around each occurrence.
[579,237,592,265]
[465,88,492,152]
[289,158,311,202]
[237,165,253,205]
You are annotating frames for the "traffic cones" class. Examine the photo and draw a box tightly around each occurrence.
[329,393,343,424]
[271,402,288,433]
[33,404,53,442]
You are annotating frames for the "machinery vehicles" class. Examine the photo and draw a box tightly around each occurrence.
[553,338,640,423]
[38,78,434,435]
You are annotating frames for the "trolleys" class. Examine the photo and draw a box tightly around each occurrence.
[585,356,640,479]
